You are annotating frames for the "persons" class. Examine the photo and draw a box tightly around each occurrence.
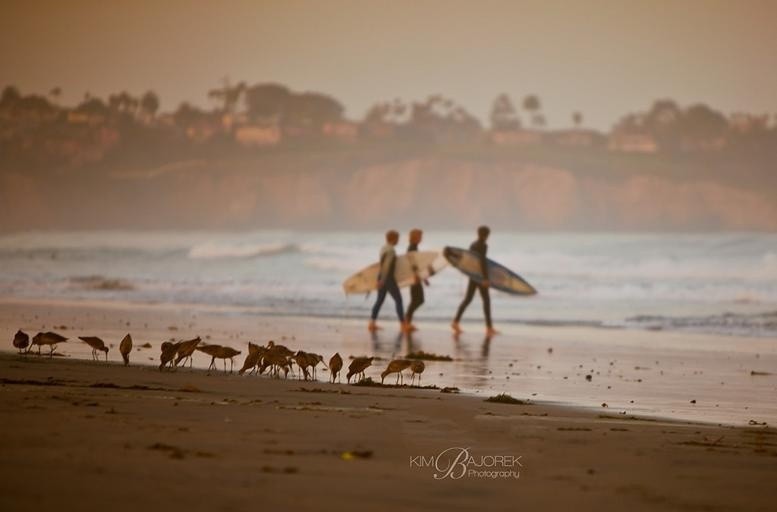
[451,226,499,335]
[369,231,407,332]
[403,229,434,330]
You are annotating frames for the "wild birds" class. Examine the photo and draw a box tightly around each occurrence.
[329,352,343,384]
[77,336,109,362]
[119,333,133,367]
[159,335,329,381]
[381,359,411,386]
[13,328,29,354]
[410,360,424,387]
[346,357,374,384]
[28,332,69,356]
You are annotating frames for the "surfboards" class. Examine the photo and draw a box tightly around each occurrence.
[443,245,537,296]
[344,246,448,295]
